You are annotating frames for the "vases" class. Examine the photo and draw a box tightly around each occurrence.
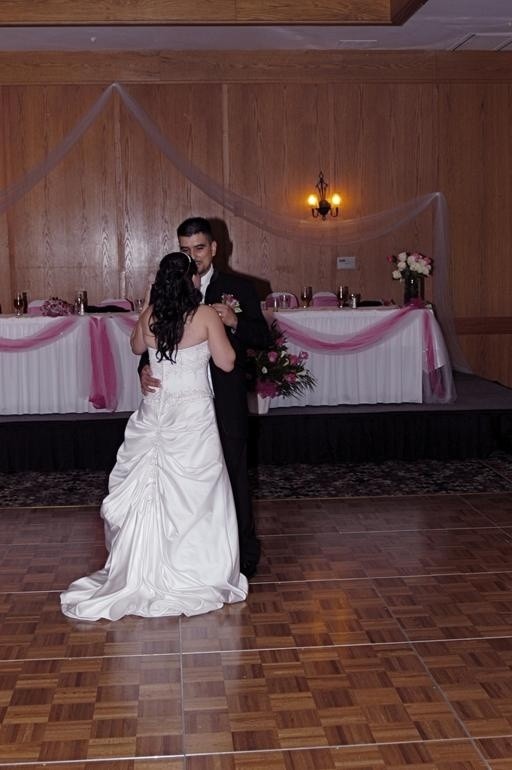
[246,392,270,416]
[403,277,421,305]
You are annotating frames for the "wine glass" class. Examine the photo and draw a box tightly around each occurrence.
[13,291,25,317]
[337,285,348,309]
[301,286,312,310]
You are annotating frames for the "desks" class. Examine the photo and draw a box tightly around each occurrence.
[2,302,439,418]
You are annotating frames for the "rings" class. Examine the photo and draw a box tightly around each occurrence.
[218,312,222,317]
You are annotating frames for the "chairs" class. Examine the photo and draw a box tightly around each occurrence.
[267,291,339,308]
[98,297,133,313]
[27,298,44,313]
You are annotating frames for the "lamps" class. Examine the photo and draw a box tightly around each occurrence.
[305,171,341,222]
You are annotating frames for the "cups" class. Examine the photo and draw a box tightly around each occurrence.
[132,287,145,314]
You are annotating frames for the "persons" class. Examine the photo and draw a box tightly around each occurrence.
[55,249,249,626]
[135,216,273,580]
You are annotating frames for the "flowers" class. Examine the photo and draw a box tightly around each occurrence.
[384,249,434,280]
[231,317,316,402]
[40,295,72,316]
